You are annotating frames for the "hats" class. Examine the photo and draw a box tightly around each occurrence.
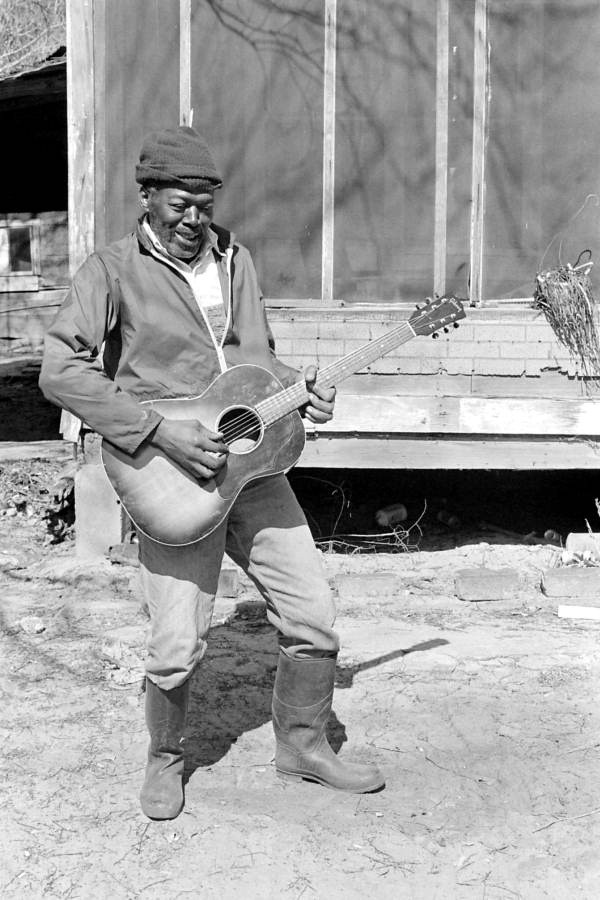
[136,125,223,193]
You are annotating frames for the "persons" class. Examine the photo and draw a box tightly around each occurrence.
[39,128,385,820]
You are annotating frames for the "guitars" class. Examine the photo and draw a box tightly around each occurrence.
[101,289,467,549]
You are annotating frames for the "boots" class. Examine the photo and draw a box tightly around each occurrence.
[272,649,385,794]
[140,675,189,820]
